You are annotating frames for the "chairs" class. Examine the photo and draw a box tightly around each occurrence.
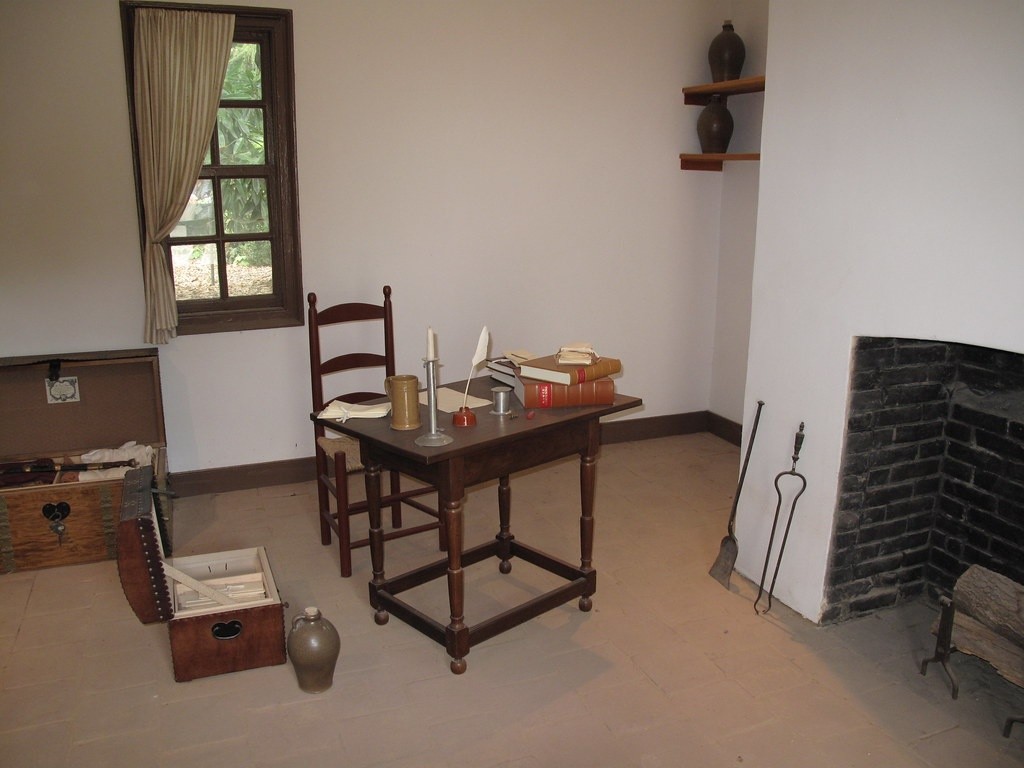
[307,284,450,578]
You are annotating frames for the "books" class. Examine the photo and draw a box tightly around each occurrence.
[487,367,515,389]
[558,343,595,365]
[518,354,621,386]
[513,368,614,408]
[486,357,518,376]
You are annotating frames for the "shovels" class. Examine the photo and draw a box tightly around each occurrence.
[708,400,765,591]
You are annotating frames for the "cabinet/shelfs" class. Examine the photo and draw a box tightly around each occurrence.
[679,75,765,171]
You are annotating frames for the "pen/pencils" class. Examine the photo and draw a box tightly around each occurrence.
[463,325,490,409]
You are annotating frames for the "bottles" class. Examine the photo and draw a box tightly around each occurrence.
[287,607,340,694]
[708,19,746,83]
[696,94,734,153]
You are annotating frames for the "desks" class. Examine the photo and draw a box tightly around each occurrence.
[311,375,643,677]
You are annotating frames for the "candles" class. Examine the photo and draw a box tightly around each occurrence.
[427,324,435,361]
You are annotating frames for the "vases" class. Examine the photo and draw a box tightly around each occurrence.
[697,96,734,153]
[708,24,746,83]
[288,606,341,695]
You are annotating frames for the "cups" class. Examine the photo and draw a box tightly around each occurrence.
[390,375,422,431]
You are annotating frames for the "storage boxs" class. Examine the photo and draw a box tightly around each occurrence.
[115,465,288,683]
[0,346,175,574]
